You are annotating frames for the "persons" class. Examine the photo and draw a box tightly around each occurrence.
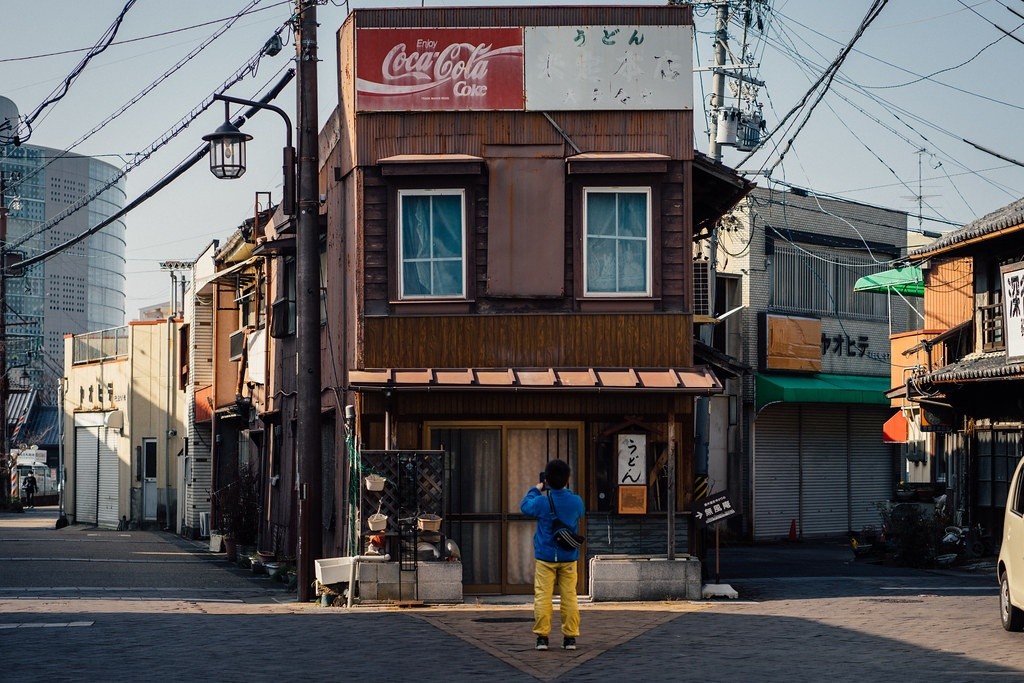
[519,460,586,651]
[22,471,39,509]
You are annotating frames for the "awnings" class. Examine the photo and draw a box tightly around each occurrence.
[852,266,927,335]
[194,256,265,297]
[754,371,892,417]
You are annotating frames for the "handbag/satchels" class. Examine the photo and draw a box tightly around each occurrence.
[547,493,585,552]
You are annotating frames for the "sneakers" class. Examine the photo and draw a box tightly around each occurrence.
[535,636,548,650]
[561,637,576,649]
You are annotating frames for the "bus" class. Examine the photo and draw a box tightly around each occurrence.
[16,462,52,498]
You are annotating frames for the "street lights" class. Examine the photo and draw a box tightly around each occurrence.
[203,2,326,602]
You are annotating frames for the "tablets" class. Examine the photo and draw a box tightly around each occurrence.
[539,472,552,491]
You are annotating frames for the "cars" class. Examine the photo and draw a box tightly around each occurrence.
[996,456,1024,630]
